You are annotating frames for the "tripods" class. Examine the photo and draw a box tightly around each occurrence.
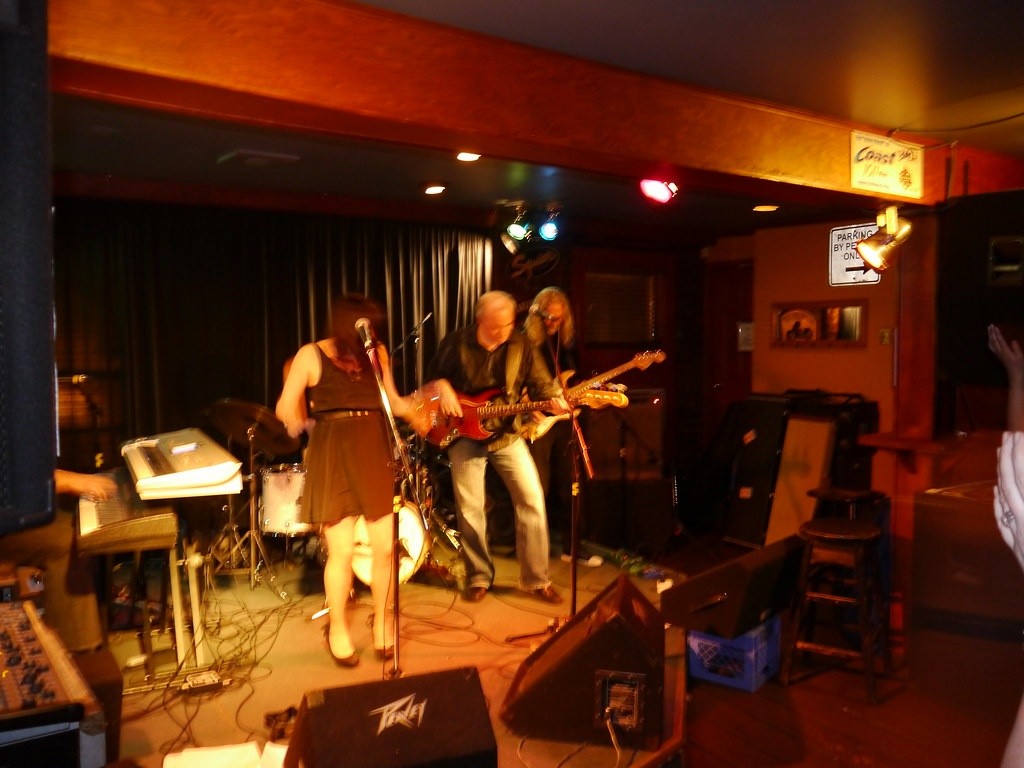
[205,425,292,605]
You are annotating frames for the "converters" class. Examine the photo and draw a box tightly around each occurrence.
[184,670,223,695]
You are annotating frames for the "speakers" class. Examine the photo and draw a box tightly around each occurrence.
[284,665,499,768]
[497,572,667,751]
[0,0,59,535]
[74,649,124,760]
[660,535,808,637]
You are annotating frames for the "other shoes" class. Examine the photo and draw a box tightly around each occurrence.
[536,584,565,605]
[465,586,488,601]
[366,614,396,658]
[321,621,359,666]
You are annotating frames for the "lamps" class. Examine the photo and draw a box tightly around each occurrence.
[500,207,535,254]
[854,204,913,273]
[539,210,564,243]
[642,179,680,205]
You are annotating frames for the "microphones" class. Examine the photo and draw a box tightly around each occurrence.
[355,318,376,354]
[529,303,559,322]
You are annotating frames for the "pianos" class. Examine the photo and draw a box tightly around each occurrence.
[76,425,244,683]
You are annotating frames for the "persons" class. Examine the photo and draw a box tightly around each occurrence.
[276,300,430,668]
[0,473,119,654]
[987,324,1024,768]
[420,288,602,605]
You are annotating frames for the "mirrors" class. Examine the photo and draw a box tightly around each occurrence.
[768,299,868,350]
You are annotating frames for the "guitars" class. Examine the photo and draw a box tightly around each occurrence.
[517,349,667,440]
[424,382,630,449]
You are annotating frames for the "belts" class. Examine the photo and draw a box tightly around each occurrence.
[313,411,384,422]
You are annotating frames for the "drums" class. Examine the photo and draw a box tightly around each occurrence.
[318,494,430,588]
[260,461,314,538]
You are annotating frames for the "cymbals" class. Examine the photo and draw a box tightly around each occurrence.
[209,399,301,456]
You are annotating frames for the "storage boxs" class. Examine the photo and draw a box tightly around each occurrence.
[687,618,783,692]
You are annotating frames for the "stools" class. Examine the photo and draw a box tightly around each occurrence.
[782,521,890,709]
[808,489,892,594]
